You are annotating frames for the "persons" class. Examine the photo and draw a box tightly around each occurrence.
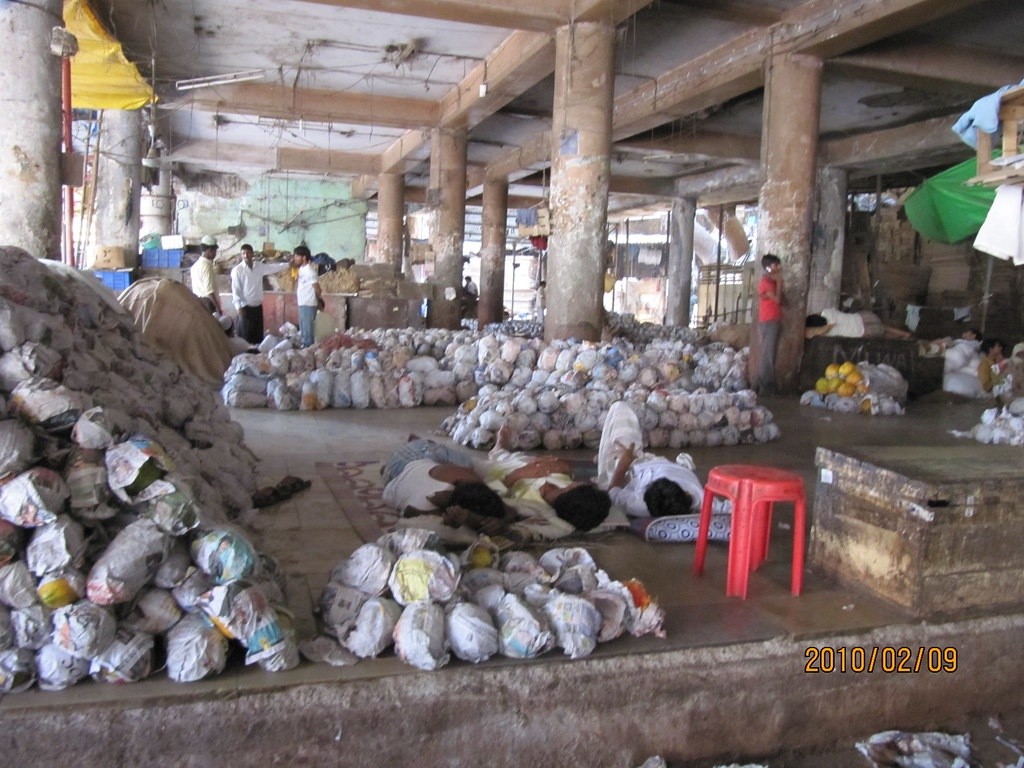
[922,329,1023,405]
[806,306,915,341]
[756,254,785,399]
[536,280,546,324]
[187,233,328,348]
[464,276,478,295]
[379,400,734,542]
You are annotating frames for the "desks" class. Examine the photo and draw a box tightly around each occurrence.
[806,444,1024,623]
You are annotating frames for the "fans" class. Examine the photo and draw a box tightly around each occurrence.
[141,50,186,168]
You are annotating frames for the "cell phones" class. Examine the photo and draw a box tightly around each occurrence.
[766,266,772,272]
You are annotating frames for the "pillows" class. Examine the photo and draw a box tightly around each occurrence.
[805,323,835,340]
[515,506,630,540]
[393,515,477,544]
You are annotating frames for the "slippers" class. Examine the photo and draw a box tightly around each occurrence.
[252,475,311,509]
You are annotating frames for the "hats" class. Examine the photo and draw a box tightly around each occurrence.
[218,316,233,330]
[202,235,216,245]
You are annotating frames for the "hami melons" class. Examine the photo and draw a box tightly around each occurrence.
[816,362,872,414]
[127,458,163,498]
[473,545,492,568]
[39,579,77,606]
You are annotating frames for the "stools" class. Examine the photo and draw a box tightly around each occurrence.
[691,464,806,600]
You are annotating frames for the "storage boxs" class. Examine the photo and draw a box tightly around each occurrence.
[160,235,186,250]
[142,247,184,269]
[94,268,134,290]
